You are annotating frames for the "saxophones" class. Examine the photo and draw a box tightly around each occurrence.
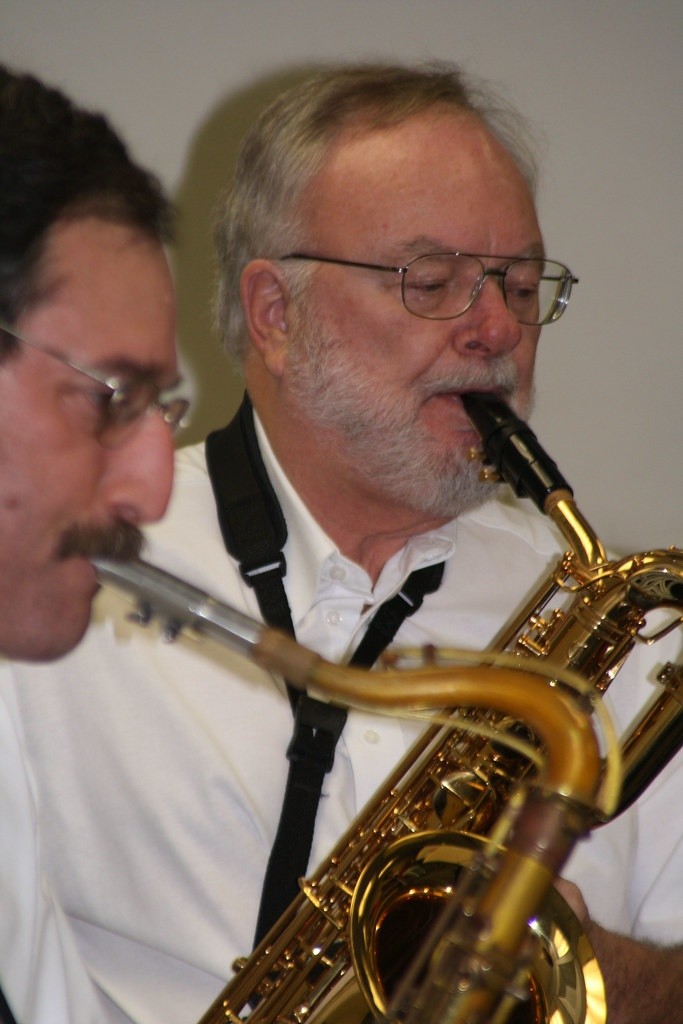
[94,551,623,1024]
[196,382,683,1024]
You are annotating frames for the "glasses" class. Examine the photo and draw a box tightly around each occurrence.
[277,252,578,326]
[0,323,190,450]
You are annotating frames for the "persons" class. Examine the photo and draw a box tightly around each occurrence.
[0,62,189,1024]
[1,62,683,1024]
